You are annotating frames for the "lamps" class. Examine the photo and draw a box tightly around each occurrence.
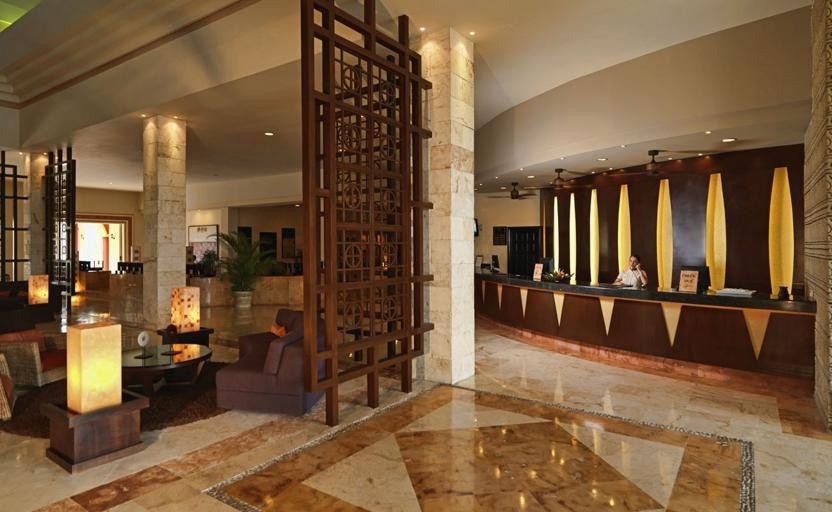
[17,272,58,329]
[153,284,216,352]
[32,318,152,476]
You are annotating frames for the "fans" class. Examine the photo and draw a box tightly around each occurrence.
[485,181,538,201]
[603,147,710,179]
[522,168,600,193]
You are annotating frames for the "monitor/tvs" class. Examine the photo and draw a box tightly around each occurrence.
[490,254,500,272]
[539,257,554,273]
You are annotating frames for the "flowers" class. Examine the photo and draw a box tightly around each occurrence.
[539,267,576,283]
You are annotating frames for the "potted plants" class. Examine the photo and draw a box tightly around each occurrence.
[202,227,281,311]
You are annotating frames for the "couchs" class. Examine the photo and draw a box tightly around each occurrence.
[0,278,57,336]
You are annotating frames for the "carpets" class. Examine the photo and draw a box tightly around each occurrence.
[1,358,236,441]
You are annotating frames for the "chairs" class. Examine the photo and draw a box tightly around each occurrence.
[212,321,332,418]
[76,260,207,280]
[236,303,323,359]
[0,326,69,391]
[0,353,20,424]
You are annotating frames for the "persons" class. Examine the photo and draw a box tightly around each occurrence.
[613,254,648,290]
